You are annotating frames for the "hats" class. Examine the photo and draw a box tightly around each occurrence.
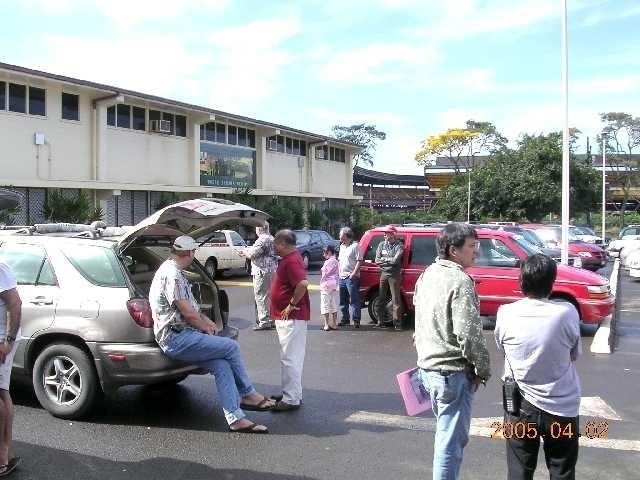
[173,235,200,252]
[382,225,397,232]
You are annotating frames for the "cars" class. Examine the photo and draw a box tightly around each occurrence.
[610,222,640,279]
[389,221,605,272]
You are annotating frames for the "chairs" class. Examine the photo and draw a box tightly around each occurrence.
[126,262,153,287]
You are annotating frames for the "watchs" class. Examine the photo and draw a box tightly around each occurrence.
[6,334,17,343]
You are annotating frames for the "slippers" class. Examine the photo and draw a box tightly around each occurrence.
[228,424,270,434]
[0,455,21,476]
[321,324,337,330]
[240,397,278,411]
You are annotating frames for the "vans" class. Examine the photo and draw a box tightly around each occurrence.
[351,226,616,326]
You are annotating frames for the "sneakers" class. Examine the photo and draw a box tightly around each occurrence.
[338,320,351,326]
[372,323,386,329]
[354,322,360,328]
[270,395,283,400]
[394,325,402,331]
[277,400,300,410]
[252,324,271,330]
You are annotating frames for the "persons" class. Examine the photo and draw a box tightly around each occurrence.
[241,219,276,333]
[373,226,408,330]
[268,228,311,413]
[337,227,366,329]
[148,235,278,433]
[318,244,342,331]
[0,251,29,478]
[494,253,581,480]
[412,222,492,480]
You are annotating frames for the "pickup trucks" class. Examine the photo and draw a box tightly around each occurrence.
[293,229,342,270]
[188,227,255,279]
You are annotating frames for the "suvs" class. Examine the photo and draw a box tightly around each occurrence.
[0,199,272,418]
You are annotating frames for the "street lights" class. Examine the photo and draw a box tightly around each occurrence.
[600,131,610,246]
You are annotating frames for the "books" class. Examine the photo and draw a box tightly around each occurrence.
[396,368,432,417]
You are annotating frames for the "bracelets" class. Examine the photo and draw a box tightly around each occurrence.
[289,301,297,307]
